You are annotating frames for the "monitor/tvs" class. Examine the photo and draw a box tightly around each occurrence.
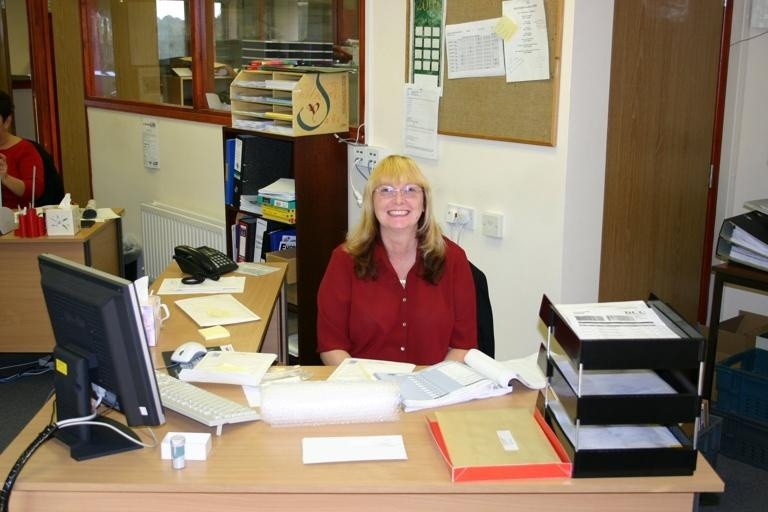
[38,253,166,461]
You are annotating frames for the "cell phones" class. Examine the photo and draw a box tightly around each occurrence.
[81,221,94,228]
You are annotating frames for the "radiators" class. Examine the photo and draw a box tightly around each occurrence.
[138,199,226,277]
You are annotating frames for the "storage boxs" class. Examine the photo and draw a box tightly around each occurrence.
[701,310,767,402]
[264,247,297,307]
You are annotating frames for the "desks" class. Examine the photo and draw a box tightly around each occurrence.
[698,260,767,414]
[0,260,726,512]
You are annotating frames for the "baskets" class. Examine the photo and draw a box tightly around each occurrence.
[691,348,767,470]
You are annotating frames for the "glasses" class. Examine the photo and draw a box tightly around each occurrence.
[374,186,422,198]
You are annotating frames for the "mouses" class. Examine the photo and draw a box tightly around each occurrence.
[83,209,97,219]
[170,342,207,364]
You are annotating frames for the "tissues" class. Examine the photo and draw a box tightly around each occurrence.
[45,192,82,236]
[135,275,161,347]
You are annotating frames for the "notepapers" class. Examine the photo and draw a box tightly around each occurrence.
[197,325,230,341]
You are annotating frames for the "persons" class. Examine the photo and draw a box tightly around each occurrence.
[317,154,480,365]
[0,91,45,208]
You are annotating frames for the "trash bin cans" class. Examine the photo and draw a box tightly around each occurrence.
[122,241,137,282]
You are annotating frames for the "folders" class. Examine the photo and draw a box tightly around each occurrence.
[260,228,297,263]
[714,210,768,273]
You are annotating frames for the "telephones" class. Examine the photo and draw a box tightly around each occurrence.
[172,245,239,277]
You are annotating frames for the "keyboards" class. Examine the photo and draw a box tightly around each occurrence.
[155,370,260,435]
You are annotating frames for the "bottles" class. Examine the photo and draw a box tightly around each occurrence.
[171,435,187,471]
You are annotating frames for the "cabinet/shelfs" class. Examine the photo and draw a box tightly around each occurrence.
[160,72,233,107]
[216,39,334,70]
[533,291,707,479]
[221,124,348,367]
[228,69,350,137]
[0,205,127,354]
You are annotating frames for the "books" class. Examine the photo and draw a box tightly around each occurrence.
[223,136,295,224]
[326,348,546,412]
[546,299,685,450]
[714,203,767,273]
[224,215,296,266]
[232,80,297,134]
